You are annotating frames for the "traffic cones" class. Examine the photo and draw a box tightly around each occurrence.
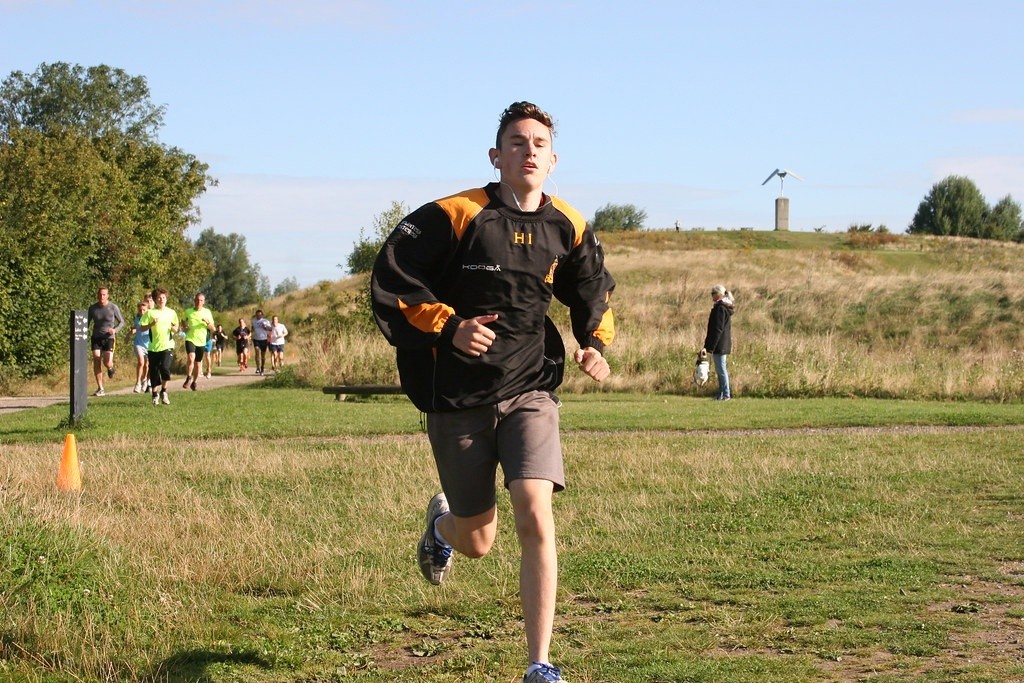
[57,432,83,495]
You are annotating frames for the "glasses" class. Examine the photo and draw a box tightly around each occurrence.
[712,292,717,294]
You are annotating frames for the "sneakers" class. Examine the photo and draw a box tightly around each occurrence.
[522,661,566,683]
[417,491,454,584]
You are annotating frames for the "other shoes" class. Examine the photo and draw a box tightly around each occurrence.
[190,381,198,391]
[160,391,170,404]
[260,369,264,376]
[94,389,105,396]
[152,393,159,406]
[256,367,260,372]
[108,367,114,377]
[218,360,221,367]
[142,381,148,391]
[134,383,141,393]
[207,369,211,378]
[183,375,191,388]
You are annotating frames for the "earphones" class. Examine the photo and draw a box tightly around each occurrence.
[548,163,552,175]
[493,158,499,169]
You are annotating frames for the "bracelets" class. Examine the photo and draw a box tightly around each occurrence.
[207,322,210,325]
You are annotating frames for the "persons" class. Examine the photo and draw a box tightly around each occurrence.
[268,315,288,369]
[87,287,123,397]
[137,288,179,404]
[213,323,228,367]
[232,317,251,368]
[370,100,615,683]
[705,285,735,401]
[204,329,212,379]
[250,309,273,375]
[182,292,215,391]
[125,293,152,393]
